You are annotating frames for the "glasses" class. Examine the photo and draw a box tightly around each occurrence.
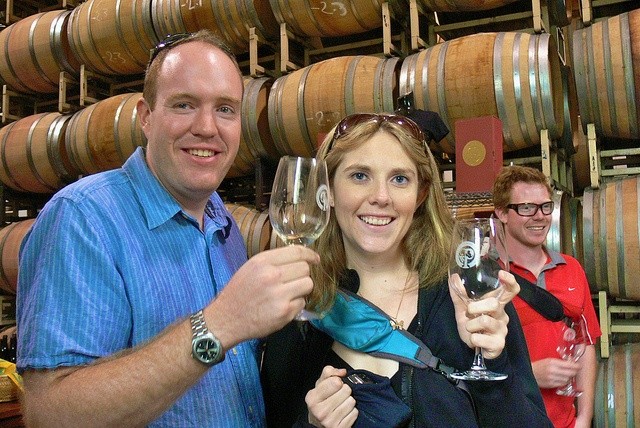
[148,31,236,71]
[323,113,425,159]
[507,202,554,217]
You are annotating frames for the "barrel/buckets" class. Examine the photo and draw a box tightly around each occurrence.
[448,191,573,258]
[593,342,640,427]
[269,203,318,250]
[68,0,159,76]
[268,55,398,157]
[0,112,77,195]
[399,32,562,156]
[220,77,272,180]
[222,202,269,260]
[581,178,639,306]
[151,1,276,56]
[571,10,640,140]
[64,93,144,177]
[1,10,80,96]
[420,0,533,15]
[0,217,36,293]
[267,0,408,38]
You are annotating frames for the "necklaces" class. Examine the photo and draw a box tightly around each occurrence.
[359,271,410,331]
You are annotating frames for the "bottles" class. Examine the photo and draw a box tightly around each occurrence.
[0,333,17,364]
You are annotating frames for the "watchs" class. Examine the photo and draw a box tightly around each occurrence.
[190,309,225,367]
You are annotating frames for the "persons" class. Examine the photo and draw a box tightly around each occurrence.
[479,167,603,428]
[258,112,554,428]
[16,29,320,427]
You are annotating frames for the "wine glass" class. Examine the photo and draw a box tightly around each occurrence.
[555,321,587,398]
[447,217,511,382]
[268,154,331,322]
